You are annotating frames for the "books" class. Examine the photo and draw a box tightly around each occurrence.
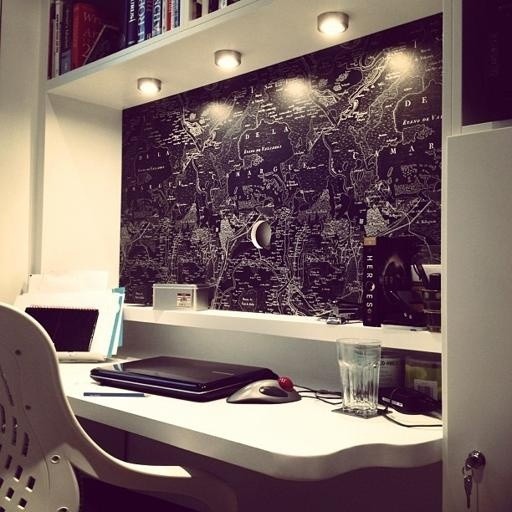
[48,0,239,79]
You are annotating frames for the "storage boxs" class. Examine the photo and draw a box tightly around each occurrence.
[151,282,209,311]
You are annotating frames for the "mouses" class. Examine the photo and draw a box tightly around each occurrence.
[226,377,301,403]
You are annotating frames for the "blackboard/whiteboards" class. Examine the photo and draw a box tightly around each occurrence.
[121,13,441,331]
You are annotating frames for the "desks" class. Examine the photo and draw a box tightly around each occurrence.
[58,354,443,511]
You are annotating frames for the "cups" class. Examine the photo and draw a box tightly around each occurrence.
[337,336,384,419]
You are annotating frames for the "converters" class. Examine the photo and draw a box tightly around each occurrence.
[379,385,433,415]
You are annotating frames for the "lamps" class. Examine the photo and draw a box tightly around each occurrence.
[136,79,162,97]
[208,220,271,307]
[215,49,242,70]
[316,10,349,36]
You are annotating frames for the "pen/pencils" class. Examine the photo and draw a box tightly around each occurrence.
[83,392,145,397]
[411,257,429,284]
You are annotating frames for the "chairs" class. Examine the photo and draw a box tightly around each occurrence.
[1,302,240,510]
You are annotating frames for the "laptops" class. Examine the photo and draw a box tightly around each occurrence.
[90,355,280,402]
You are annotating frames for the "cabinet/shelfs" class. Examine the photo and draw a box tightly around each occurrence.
[39,1,263,90]
